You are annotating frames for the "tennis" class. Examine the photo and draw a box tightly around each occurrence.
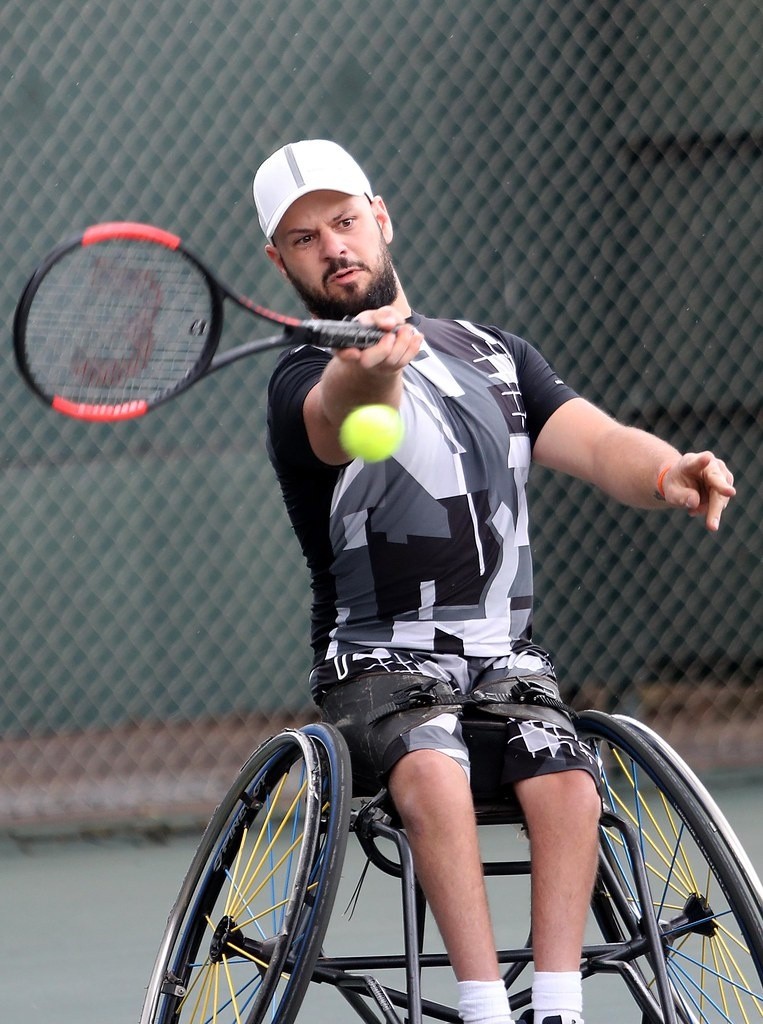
[336,403,405,463]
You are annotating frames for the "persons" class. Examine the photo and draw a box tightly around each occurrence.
[252,140,738,1024]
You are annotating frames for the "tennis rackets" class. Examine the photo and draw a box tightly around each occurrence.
[10,218,397,425]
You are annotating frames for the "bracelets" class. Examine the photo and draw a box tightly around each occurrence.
[657,460,675,500]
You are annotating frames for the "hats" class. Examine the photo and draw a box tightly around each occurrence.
[253,139,374,248]
